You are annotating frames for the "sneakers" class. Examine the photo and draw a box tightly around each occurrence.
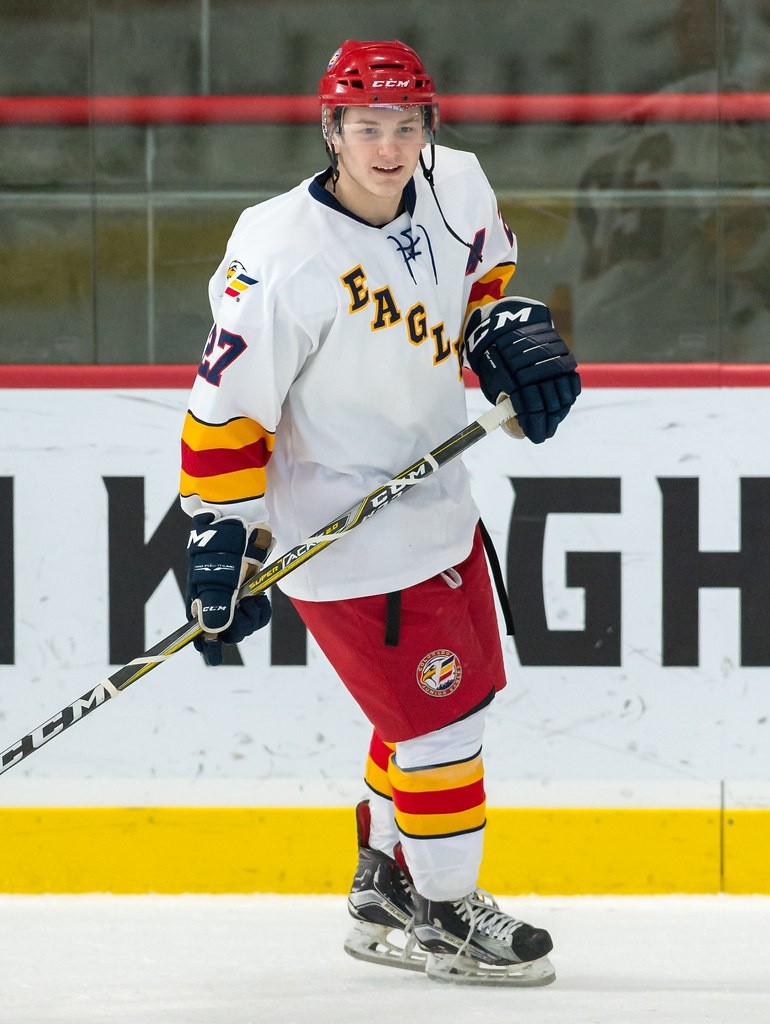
[393,839,556,988]
[343,799,501,971]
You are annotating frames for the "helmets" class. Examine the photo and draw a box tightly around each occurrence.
[318,39,437,136]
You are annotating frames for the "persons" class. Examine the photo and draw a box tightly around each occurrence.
[179,38,582,986]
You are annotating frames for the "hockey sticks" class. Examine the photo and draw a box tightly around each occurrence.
[1,395,517,774]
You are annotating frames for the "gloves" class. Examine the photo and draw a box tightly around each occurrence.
[185,509,277,666]
[463,297,582,444]
